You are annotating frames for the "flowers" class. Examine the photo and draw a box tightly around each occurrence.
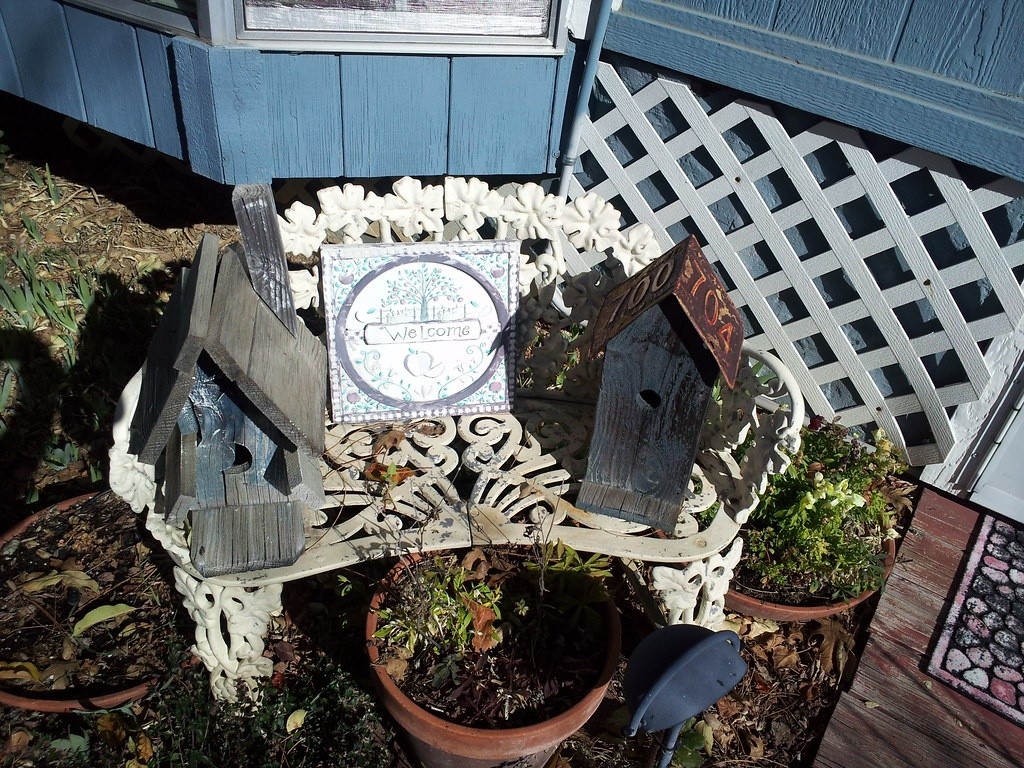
[723,412,915,594]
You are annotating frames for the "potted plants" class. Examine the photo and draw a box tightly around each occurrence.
[365,544,624,767]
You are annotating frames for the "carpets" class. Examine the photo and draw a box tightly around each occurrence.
[920,509,1024,730]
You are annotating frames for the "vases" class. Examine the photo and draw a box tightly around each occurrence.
[0,490,205,713]
[718,491,898,622]
